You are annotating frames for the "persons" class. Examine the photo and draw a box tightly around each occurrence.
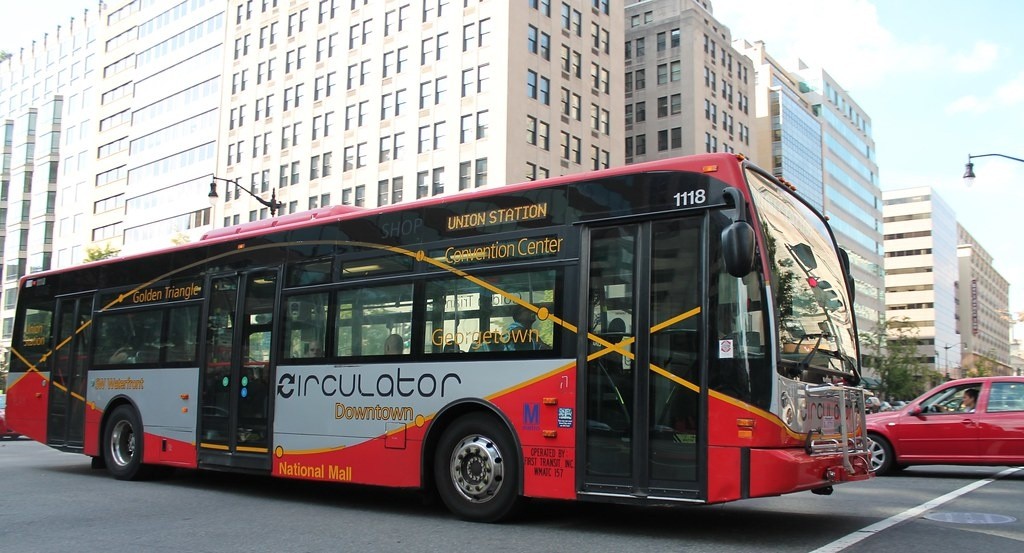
[501,305,551,350]
[308,339,322,357]
[444,341,460,353]
[384,334,403,355]
[117,334,192,363]
[929,387,980,412]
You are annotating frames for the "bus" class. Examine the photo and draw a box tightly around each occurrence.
[5,150,872,524]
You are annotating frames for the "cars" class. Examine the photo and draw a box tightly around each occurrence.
[0,394,22,439]
[201,403,267,444]
[864,376,1024,478]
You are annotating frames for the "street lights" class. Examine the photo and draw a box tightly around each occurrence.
[944,342,967,383]
